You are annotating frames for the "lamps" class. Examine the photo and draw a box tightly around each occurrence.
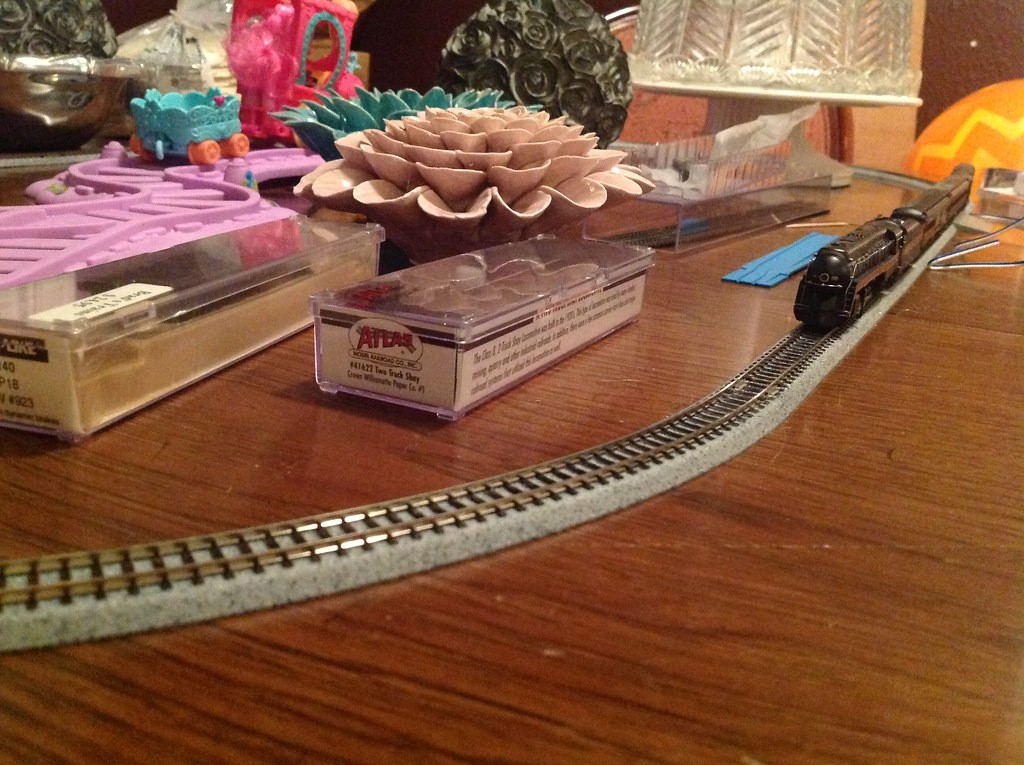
[627,0,929,188]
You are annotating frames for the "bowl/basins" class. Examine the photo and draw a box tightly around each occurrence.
[0,70,128,150]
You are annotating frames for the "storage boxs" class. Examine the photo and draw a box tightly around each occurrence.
[308,232,658,420]
[0,214,389,444]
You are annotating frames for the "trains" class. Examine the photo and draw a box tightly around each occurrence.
[793,161,975,325]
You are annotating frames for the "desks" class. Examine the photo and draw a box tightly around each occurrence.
[0,176,1024,765]
